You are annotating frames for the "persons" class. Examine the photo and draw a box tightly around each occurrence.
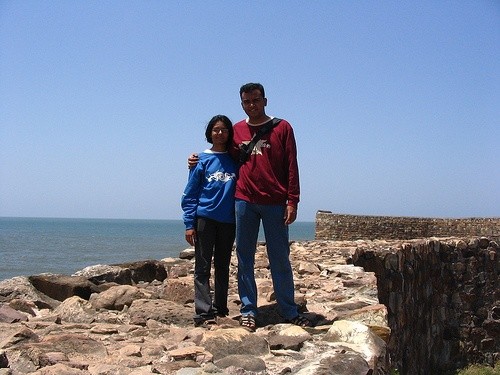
[181,114,238,324]
[189,83,313,331]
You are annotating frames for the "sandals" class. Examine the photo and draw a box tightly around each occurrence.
[240,316,256,332]
[290,316,313,327]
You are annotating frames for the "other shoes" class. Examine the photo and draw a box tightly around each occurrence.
[195,320,219,328]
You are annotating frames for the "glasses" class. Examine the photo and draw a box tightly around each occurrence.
[213,128,229,133]
